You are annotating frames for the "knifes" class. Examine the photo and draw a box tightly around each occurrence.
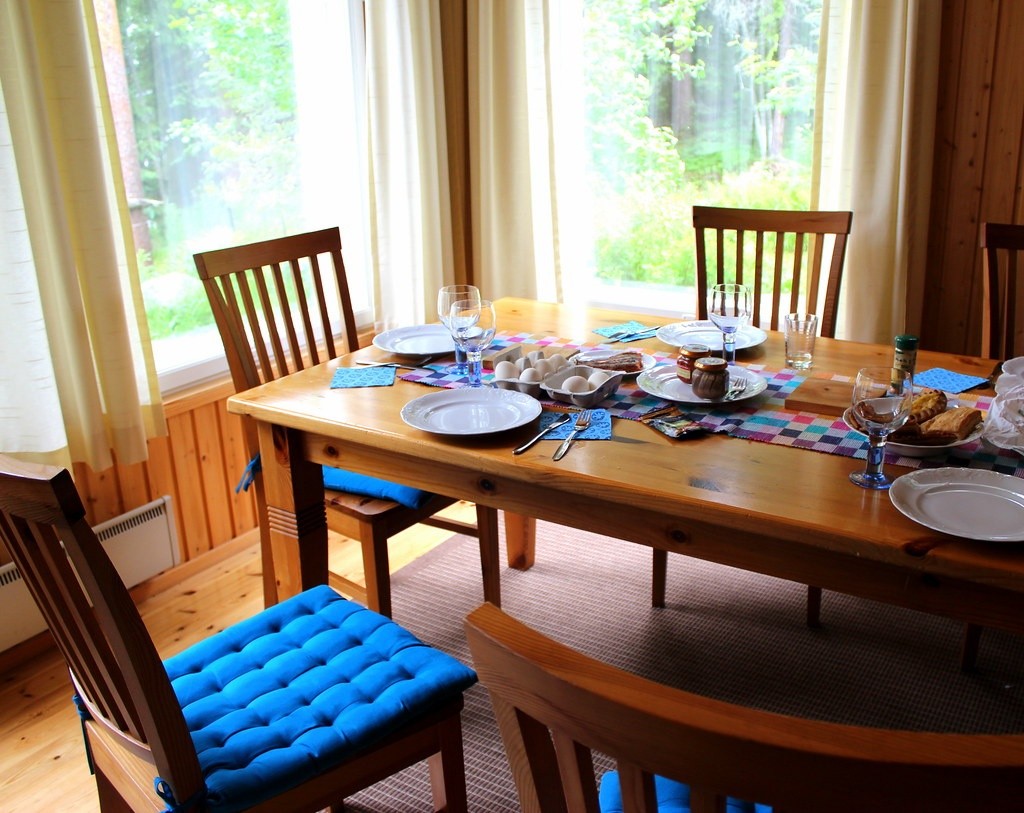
[357,359,437,373]
[602,326,660,343]
[512,413,571,455]
[977,361,1004,390]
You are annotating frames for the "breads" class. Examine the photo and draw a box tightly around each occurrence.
[846,388,983,444]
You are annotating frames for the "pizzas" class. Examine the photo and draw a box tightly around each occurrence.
[576,351,643,372]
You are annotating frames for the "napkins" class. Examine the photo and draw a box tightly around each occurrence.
[537,408,613,442]
[594,319,658,344]
[912,366,990,394]
[330,364,396,389]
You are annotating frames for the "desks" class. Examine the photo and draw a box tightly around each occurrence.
[227,297,1024,698]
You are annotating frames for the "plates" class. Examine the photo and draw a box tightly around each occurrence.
[568,349,656,376]
[1002,357,1024,380]
[889,467,1024,541]
[400,387,542,437]
[372,323,483,357]
[843,396,985,458]
[656,320,768,352]
[636,364,768,405]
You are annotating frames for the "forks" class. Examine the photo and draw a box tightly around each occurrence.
[353,355,433,369]
[552,408,592,461]
[722,377,747,401]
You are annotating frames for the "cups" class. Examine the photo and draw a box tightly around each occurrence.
[783,313,818,371]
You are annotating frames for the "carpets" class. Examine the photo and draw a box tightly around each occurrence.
[318,508,1024,813]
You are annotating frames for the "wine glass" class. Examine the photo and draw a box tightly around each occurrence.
[848,367,913,490]
[438,285,481,376]
[449,299,497,389]
[708,284,752,366]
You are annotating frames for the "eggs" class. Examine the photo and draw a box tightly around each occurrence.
[495,351,568,383]
[562,371,609,393]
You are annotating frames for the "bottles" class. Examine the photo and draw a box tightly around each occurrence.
[676,343,730,400]
[885,335,919,398]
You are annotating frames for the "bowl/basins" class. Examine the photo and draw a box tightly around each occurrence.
[489,361,626,408]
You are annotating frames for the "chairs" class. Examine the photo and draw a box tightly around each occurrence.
[1,455,478,813]
[651,202,852,628]
[459,601,1024,813]
[193,227,500,620]
[958,218,1024,673]
